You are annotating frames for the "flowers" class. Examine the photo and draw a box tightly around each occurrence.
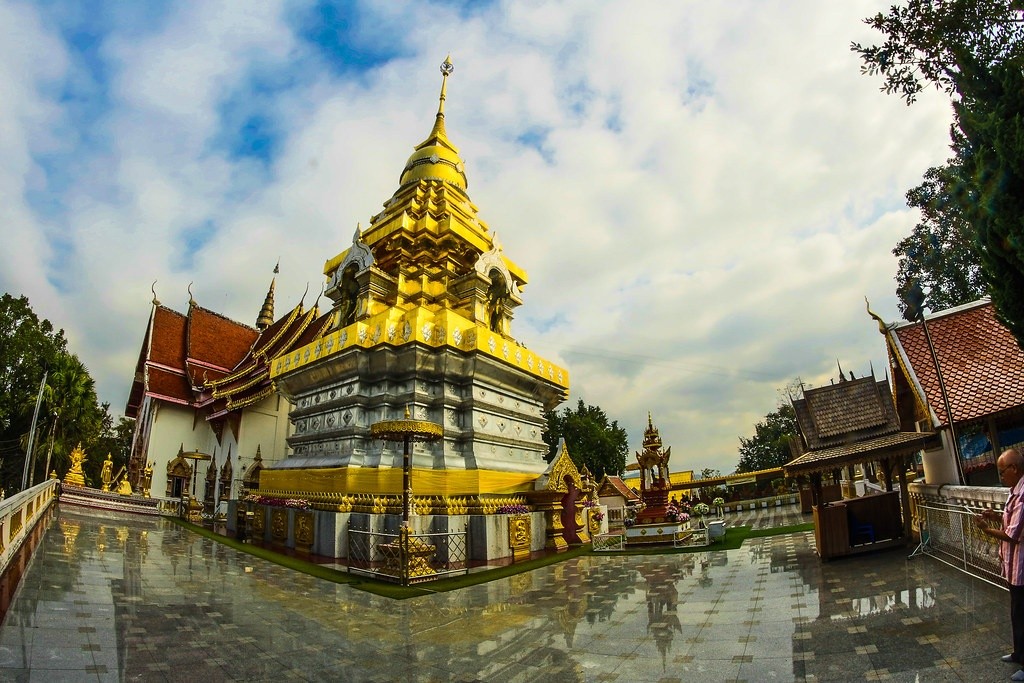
[666,507,679,518]
[677,513,691,521]
[681,502,691,509]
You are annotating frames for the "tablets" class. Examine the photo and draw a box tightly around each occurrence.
[964,506,989,527]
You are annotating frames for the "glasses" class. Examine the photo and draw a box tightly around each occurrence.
[998,464,1015,475]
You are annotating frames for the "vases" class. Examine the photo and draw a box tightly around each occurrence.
[681,520,686,523]
[671,517,677,523]
[683,507,688,511]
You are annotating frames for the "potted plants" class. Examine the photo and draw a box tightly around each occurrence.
[713,497,725,518]
[693,503,709,529]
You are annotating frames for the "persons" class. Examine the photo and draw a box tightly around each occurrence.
[100,451,113,486]
[142,461,153,492]
[671,495,699,507]
[68,456,83,474]
[973,449,1024,682]
[119,475,132,496]
